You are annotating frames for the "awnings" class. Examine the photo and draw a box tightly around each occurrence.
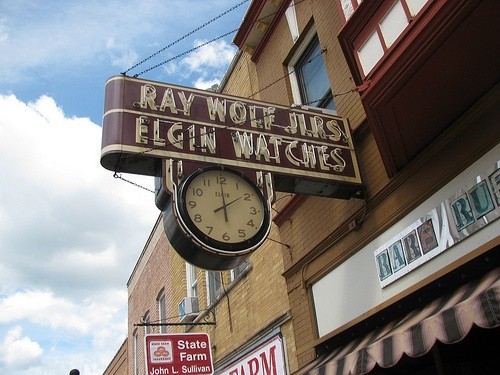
[289,265,500,375]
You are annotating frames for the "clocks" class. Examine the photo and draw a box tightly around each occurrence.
[155,157,276,272]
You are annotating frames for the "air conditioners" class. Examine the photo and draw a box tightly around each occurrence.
[177,296,198,325]
